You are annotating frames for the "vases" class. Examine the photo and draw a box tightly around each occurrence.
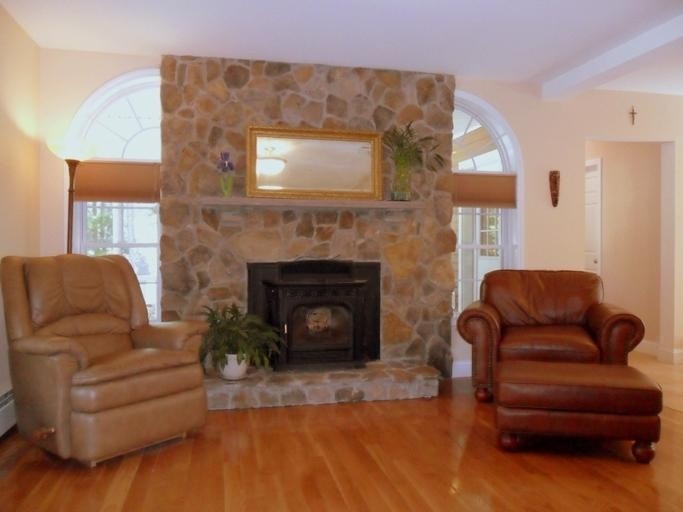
[215,149,237,172]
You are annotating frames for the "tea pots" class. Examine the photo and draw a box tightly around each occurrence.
[243,122,383,199]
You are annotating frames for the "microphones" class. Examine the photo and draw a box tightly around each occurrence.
[488,358,665,465]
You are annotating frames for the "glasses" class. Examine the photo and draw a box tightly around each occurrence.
[546,168,562,208]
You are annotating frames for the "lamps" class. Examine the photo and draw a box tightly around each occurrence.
[455,267,646,404]
[1,251,206,471]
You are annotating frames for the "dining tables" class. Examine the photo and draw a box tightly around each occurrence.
[198,299,280,381]
[380,121,445,202]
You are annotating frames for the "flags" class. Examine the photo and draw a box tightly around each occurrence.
[218,171,234,196]
